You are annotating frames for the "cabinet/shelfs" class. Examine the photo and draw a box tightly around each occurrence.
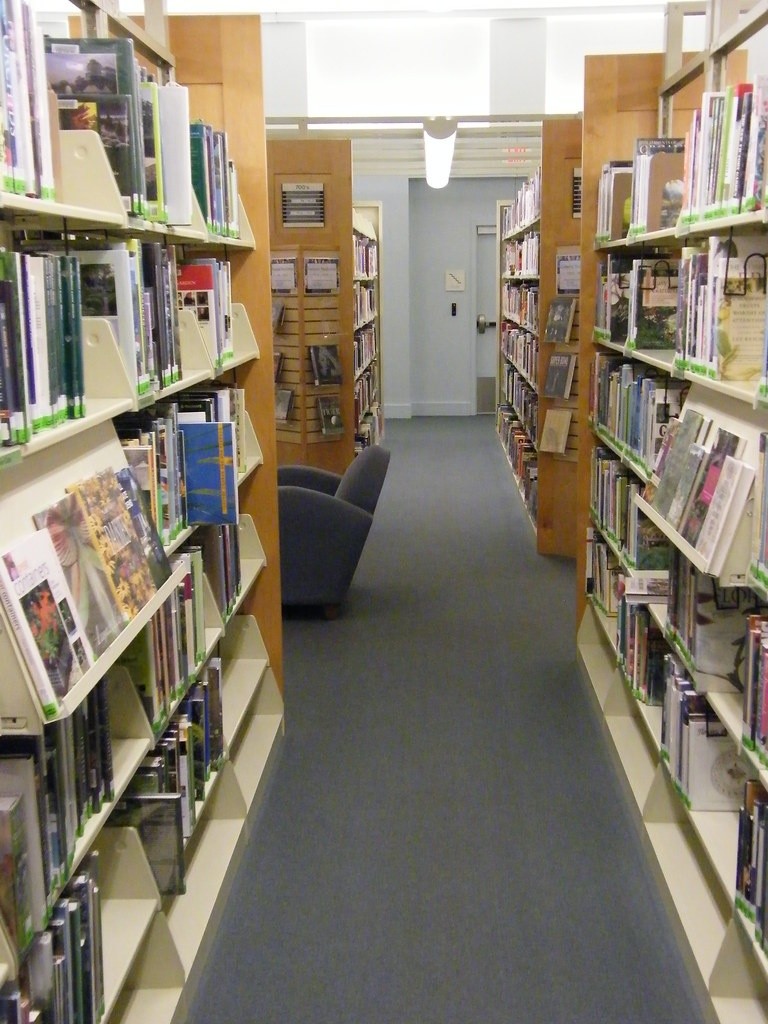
[1,4,766,1024]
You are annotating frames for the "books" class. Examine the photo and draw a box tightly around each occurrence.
[541,295,576,345]
[578,64,767,970]
[538,408,572,454]
[498,165,539,518]
[542,352,578,400]
[1,0,244,1024]
[270,227,387,454]
